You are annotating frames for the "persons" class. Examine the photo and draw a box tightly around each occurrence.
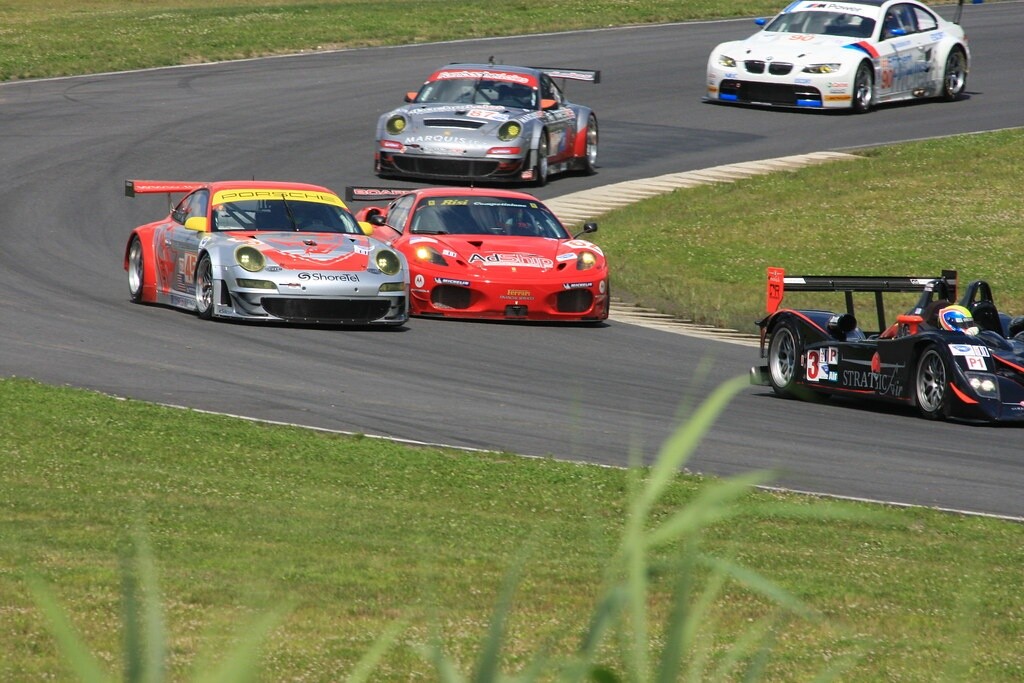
[937,305,980,336]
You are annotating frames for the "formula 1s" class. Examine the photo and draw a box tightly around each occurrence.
[749,267,1024,427]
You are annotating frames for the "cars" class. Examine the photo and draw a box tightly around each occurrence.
[702,0,973,114]
[122,179,413,330]
[374,56,602,191]
[364,188,613,326]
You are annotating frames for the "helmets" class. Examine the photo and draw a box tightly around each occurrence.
[286,202,308,225]
[499,206,523,225]
[938,305,973,333]
[513,83,531,105]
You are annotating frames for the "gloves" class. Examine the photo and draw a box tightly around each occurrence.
[965,327,979,337]
